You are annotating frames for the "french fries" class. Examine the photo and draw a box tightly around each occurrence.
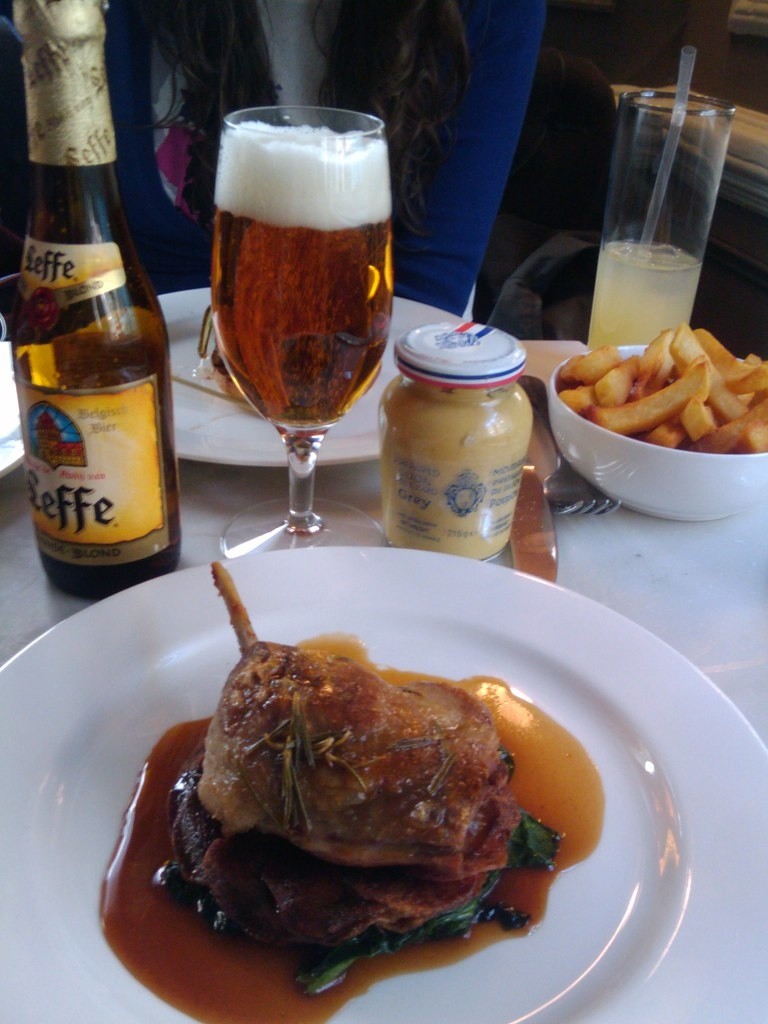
[558,322,767,454]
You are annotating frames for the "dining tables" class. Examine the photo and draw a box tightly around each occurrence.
[0,286,768,752]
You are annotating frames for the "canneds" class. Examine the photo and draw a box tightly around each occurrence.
[377,321,531,562]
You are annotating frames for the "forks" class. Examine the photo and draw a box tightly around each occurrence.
[516,374,621,515]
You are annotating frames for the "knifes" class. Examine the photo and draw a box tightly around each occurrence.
[511,459,557,586]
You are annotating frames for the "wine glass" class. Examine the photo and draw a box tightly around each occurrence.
[208,105,392,553]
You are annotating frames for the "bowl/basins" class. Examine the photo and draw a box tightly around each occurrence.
[548,344,768,521]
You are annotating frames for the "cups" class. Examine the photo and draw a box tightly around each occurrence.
[585,90,737,353]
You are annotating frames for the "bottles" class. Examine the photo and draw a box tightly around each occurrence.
[9,0,182,597]
[380,322,534,562]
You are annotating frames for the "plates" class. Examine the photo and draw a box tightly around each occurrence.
[0,548,768,1019]
[159,286,465,464]
[1,345,26,481]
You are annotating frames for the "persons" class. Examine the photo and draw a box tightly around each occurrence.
[0,0,546,318]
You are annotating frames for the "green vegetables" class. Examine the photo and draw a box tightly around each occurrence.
[160,747,560,997]
